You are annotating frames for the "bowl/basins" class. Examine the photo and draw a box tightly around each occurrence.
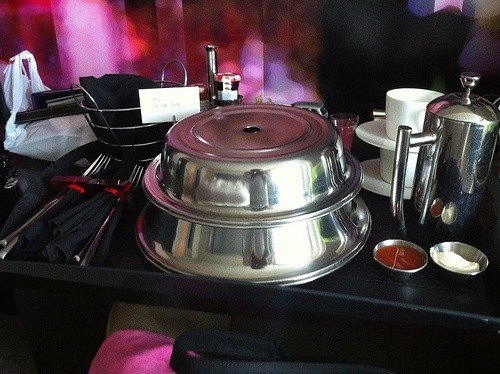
[429,241,489,285]
[371,238,428,282]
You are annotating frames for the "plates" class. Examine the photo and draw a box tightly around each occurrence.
[355,119,420,152]
[359,159,412,200]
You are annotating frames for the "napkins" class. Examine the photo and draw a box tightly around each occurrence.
[46,171,140,261]
[0,158,120,260]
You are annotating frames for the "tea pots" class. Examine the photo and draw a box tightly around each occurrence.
[389,71,500,242]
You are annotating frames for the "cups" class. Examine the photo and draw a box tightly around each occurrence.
[385,88,445,143]
[330,112,359,152]
[379,148,418,188]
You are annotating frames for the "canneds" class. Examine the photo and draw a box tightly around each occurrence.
[215,72,242,100]
[190,83,209,111]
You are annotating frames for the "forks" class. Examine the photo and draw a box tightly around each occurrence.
[79,164,144,266]
[0,154,111,245]
